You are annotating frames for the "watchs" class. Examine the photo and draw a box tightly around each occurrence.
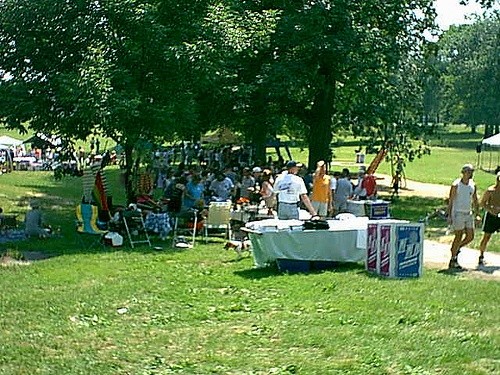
[476,215,482,220]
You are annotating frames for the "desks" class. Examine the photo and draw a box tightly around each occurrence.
[241,219,410,268]
[347,199,390,219]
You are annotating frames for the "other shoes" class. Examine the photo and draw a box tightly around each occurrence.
[479,256,484,265]
[451,248,460,255]
[449,262,462,269]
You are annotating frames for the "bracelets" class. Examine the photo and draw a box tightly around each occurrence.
[371,193,375,194]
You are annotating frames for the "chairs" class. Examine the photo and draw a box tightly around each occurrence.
[75,197,237,250]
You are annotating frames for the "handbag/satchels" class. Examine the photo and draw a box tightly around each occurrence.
[304,215,329,229]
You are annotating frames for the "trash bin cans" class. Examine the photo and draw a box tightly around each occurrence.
[357,154,364,164]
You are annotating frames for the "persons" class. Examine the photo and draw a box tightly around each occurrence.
[447,164,482,269]
[478,165,500,265]
[54,166,62,180]
[0,137,117,169]
[119,143,378,236]
[391,171,402,199]
[24,199,50,239]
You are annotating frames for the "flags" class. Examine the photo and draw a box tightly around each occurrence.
[91,172,112,222]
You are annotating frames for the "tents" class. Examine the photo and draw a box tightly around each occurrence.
[0,135,25,149]
[479,133,500,172]
[22,133,64,146]
[200,122,296,162]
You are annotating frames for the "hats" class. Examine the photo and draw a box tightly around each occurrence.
[30,199,40,208]
[253,167,261,172]
[463,164,475,170]
[286,160,300,168]
